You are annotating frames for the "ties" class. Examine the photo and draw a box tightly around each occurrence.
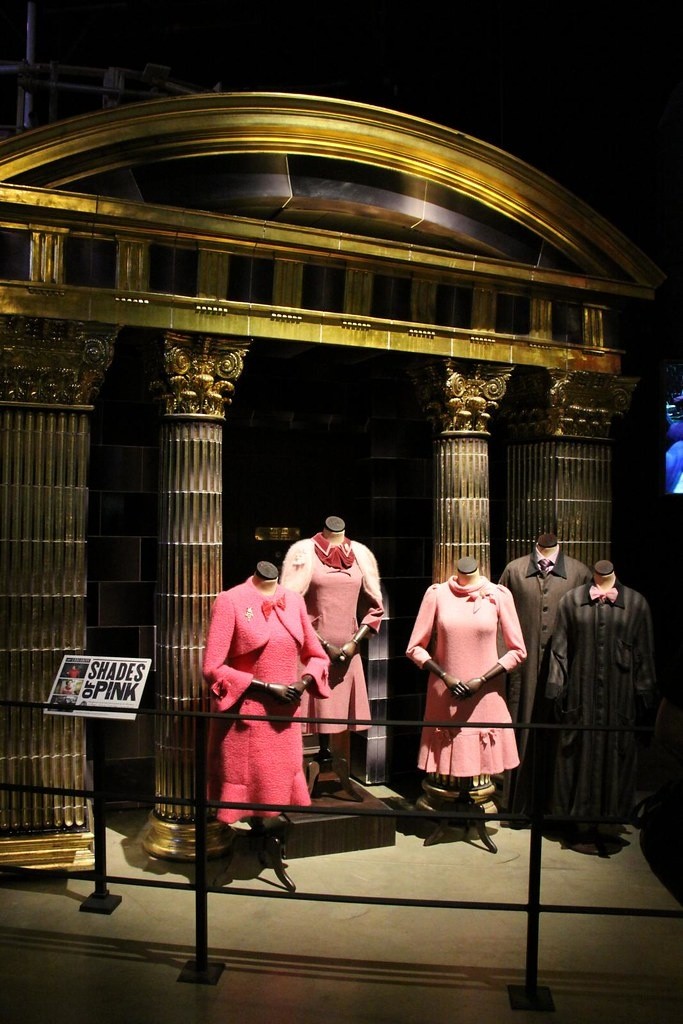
[539,559,552,576]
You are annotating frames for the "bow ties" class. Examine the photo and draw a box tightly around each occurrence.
[589,585,618,603]
[261,596,285,619]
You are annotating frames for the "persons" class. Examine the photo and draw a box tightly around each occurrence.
[280,517,384,801]
[203,561,329,893]
[547,561,656,855]
[405,556,526,853]
[497,534,592,831]
